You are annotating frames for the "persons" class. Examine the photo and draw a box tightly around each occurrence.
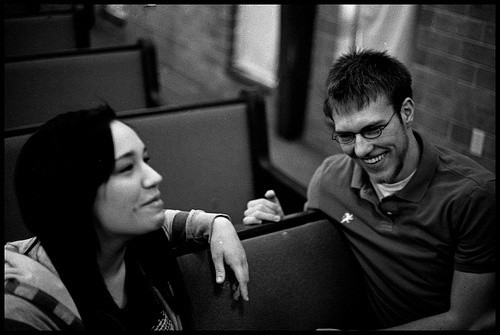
[4,104,249,331]
[242,50,495,330]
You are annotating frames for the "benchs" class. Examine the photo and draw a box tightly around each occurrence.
[3,37,160,128]
[4,89,272,242]
[172,209,363,332]
[3,8,90,56]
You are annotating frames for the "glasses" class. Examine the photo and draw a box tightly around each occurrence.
[331,108,397,144]
